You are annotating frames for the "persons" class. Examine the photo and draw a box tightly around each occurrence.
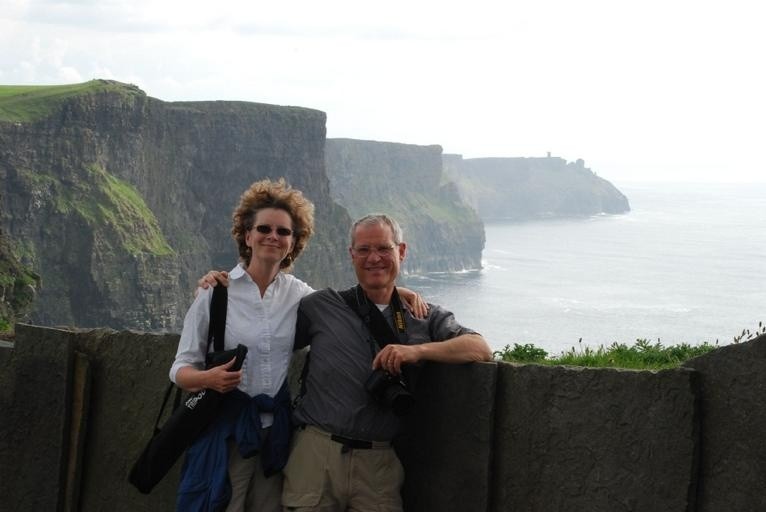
[192,214,492,512]
[169,177,429,512]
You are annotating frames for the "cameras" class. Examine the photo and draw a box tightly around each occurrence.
[365,368,417,412]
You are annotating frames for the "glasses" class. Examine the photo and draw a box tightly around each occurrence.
[248,224,296,236]
[353,243,397,258]
